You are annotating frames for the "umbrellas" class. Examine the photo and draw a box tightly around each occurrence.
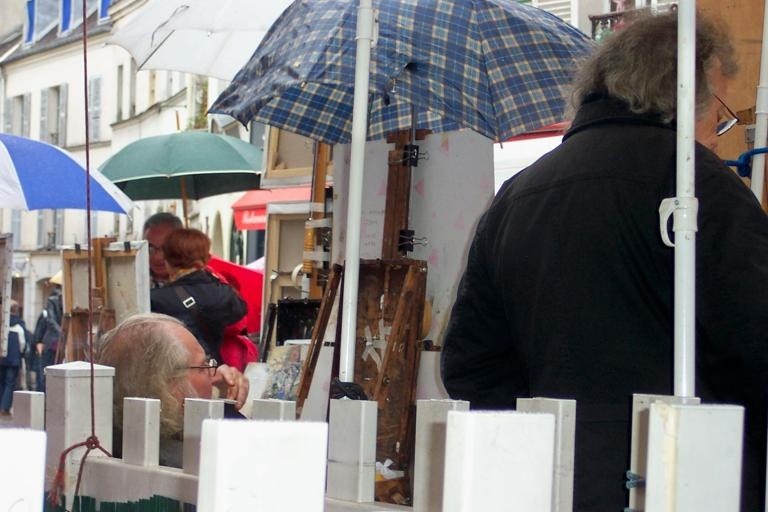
[0,134,140,215]
[103,0,294,83]
[97,131,264,201]
[204,0,608,151]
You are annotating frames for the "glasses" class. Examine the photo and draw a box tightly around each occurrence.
[149,243,163,256]
[714,93,740,137]
[188,358,219,376]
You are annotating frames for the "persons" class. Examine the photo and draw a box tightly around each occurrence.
[34,270,64,391]
[438,5,768,511]
[97,312,250,469]
[1,300,26,417]
[150,228,248,369]
[142,212,183,290]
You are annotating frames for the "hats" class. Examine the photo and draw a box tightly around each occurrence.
[50,271,63,286]
